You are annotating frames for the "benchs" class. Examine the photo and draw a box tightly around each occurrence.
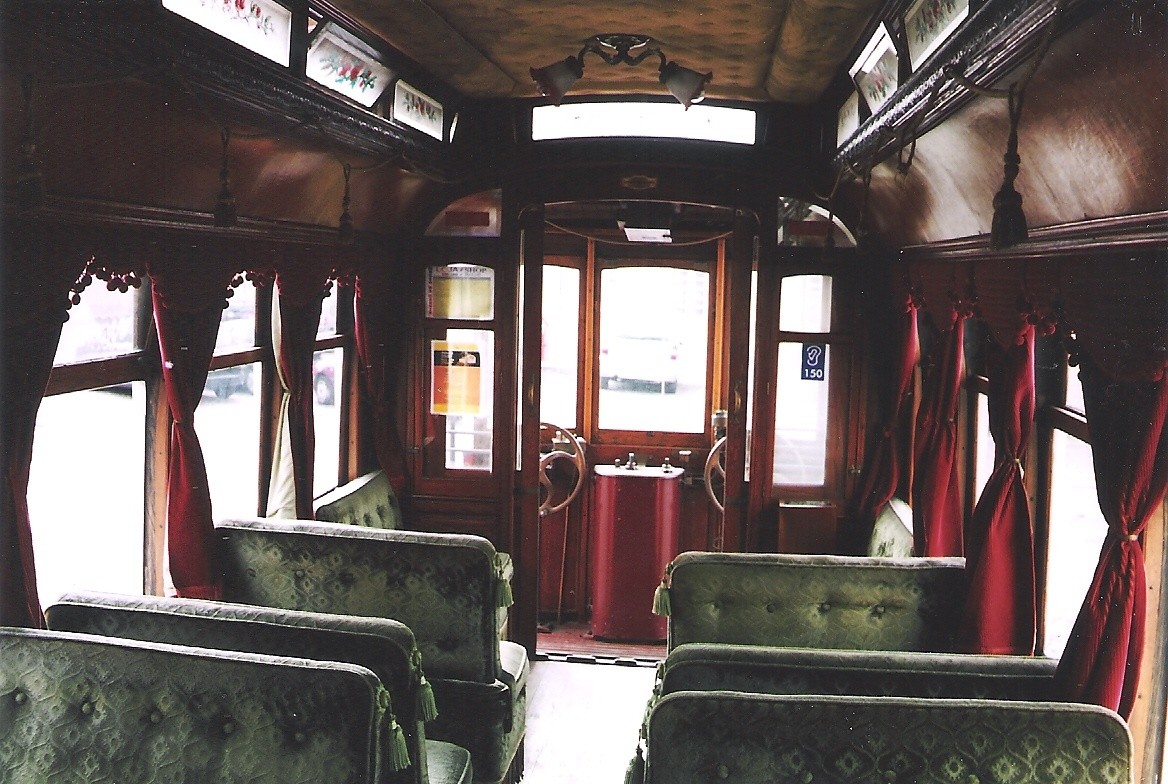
[0,471,533,784]
[624,498,1132,784]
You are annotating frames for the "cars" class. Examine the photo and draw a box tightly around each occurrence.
[203,321,256,399]
[312,350,334,406]
[600,307,683,394]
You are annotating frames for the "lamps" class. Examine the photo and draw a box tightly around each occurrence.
[528,36,716,112]
